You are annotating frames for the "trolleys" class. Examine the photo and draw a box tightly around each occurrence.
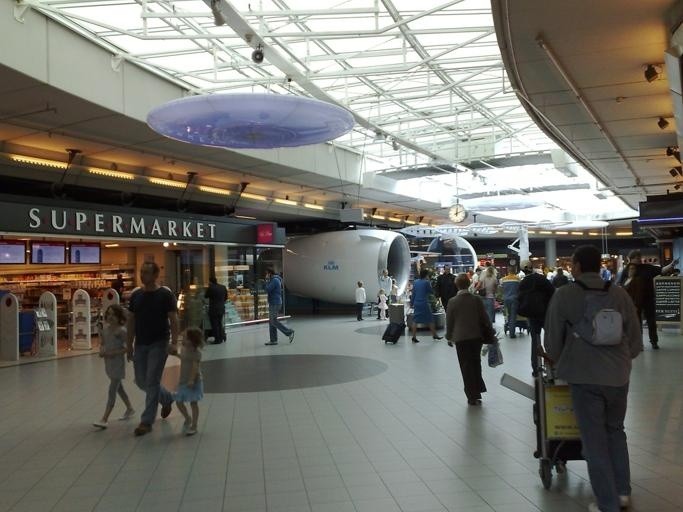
[504,309,530,336]
[533,323,590,490]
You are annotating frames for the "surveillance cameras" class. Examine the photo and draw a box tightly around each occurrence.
[286,75,293,82]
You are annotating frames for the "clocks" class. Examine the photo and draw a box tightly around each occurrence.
[448,203,467,223]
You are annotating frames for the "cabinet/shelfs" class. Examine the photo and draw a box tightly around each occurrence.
[0,263,134,338]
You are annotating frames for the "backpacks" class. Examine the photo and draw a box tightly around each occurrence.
[567,279,623,347]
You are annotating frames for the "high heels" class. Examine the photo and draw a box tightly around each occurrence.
[412,336,419,343]
[433,336,444,340]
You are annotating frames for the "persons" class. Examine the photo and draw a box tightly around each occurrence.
[111,274,123,296]
[127,262,181,435]
[204,277,226,344]
[172,326,204,435]
[94,304,135,429]
[263,268,294,345]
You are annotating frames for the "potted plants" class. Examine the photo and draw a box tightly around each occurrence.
[405,265,445,333]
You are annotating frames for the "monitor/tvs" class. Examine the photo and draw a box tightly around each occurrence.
[68,242,101,264]
[0,239,27,264]
[29,240,66,264]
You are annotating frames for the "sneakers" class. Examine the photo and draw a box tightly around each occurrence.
[264,341,278,344]
[160,393,176,418]
[91,420,108,428]
[288,331,294,343]
[134,422,151,435]
[181,415,198,435]
[119,409,134,420]
[588,496,630,512]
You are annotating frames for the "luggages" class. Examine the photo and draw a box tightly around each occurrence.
[382,307,414,344]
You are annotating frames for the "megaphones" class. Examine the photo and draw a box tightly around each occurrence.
[252,50,263,63]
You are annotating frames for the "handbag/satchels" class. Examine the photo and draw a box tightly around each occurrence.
[479,288,486,296]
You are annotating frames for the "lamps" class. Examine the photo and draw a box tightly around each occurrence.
[641,59,682,189]
